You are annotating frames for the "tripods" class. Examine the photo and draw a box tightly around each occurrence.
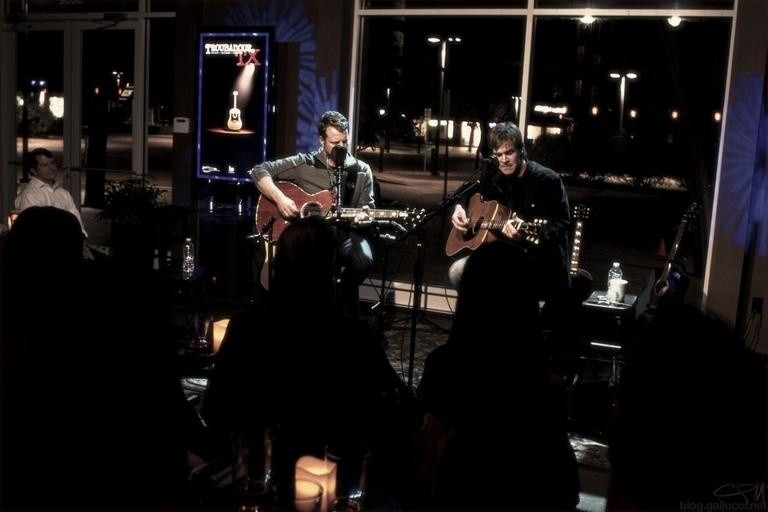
[392,235,450,333]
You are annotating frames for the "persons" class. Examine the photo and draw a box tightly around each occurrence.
[1,110,768,511]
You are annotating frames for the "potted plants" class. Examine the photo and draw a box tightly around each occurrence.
[95,173,166,259]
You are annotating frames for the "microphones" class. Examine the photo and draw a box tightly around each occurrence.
[479,154,499,176]
[262,215,277,234]
[331,145,349,165]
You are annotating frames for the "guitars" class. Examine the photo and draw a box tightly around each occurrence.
[636,205,700,321]
[445,194,547,260]
[540,204,592,330]
[256,179,423,245]
[227,90,243,130]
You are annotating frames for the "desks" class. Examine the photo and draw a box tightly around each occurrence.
[93,224,272,314]
[193,462,405,512]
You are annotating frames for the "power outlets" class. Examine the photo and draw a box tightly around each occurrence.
[751,298,762,313]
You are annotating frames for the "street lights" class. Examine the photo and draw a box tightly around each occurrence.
[426,34,463,179]
[607,71,641,146]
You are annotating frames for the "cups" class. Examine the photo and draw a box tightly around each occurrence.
[609,278,629,303]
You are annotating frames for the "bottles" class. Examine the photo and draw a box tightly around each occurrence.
[608,260,623,293]
[152,248,161,271]
[180,235,195,279]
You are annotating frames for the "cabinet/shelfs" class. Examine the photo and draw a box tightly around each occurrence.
[559,289,639,499]
